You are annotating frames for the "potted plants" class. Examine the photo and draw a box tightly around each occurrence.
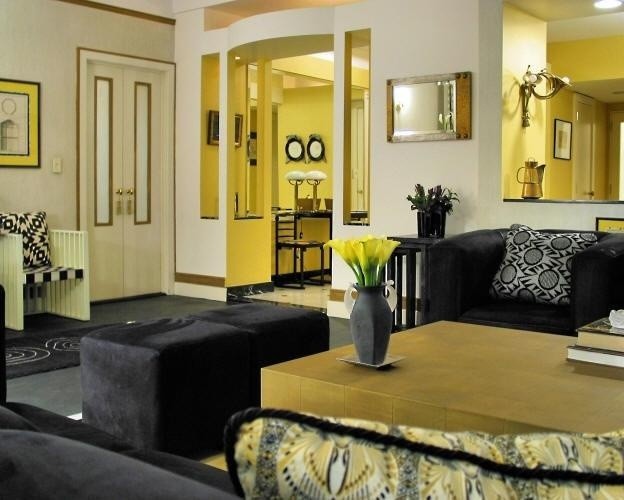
[407,183,461,239]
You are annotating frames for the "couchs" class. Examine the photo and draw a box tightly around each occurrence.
[0,397,623,500]
[80,317,258,461]
[427,223,624,336]
[184,302,329,414]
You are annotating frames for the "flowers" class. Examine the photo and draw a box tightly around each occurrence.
[322,235,402,286]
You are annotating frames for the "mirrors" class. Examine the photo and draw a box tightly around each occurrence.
[245,61,370,225]
[386,71,473,144]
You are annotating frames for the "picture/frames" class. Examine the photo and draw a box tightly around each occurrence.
[595,217,624,234]
[208,111,243,148]
[304,134,329,164]
[250,132,256,166]
[1,78,41,169]
[283,134,305,164]
[552,118,573,161]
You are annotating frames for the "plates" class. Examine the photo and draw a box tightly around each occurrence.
[337,351,406,369]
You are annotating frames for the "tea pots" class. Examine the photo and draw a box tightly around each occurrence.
[516,157,546,200]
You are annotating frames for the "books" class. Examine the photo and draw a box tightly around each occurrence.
[565,317,624,368]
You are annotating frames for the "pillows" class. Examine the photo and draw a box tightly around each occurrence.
[490,224,598,307]
[222,407,624,500]
[0,208,53,268]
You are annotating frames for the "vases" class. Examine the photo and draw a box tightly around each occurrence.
[349,283,394,365]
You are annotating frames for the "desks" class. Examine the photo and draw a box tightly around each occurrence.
[293,211,368,285]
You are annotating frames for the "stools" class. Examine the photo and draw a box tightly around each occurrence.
[386,234,449,333]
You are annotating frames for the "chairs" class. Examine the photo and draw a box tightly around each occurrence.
[0,208,91,331]
[274,213,325,290]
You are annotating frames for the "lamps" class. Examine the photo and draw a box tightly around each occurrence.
[306,171,327,211]
[286,171,306,209]
[519,64,573,129]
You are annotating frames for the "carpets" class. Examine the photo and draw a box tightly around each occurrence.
[5,322,132,381]
